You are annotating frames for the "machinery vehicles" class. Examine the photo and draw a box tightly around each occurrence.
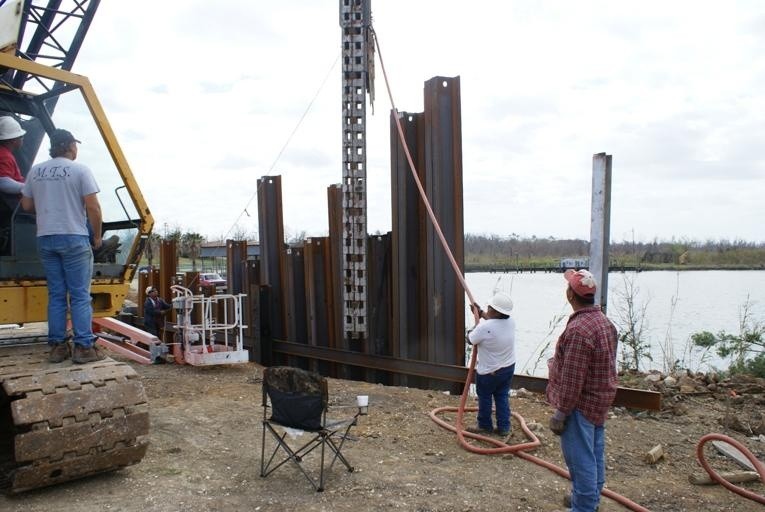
[0,0,166,494]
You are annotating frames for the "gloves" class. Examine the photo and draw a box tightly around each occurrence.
[549,409,567,436]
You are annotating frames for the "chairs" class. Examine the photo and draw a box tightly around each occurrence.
[258,367,369,494]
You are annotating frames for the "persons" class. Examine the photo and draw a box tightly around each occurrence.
[20,129,106,363]
[0,115,26,212]
[464,293,516,437]
[144,285,172,363]
[545,266,618,512]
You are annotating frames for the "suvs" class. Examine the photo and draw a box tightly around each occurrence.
[199,272,229,293]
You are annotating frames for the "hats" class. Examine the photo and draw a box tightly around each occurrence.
[144,287,155,296]
[487,293,514,317]
[49,128,82,149]
[0,115,27,140]
[563,268,598,301]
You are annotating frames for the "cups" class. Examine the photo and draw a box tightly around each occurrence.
[356,395,369,414]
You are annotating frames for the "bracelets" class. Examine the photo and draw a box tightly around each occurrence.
[479,309,483,317]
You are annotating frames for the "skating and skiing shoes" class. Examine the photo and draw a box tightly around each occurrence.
[47,340,71,363]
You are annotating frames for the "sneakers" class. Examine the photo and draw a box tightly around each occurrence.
[464,424,488,433]
[562,494,600,512]
[72,342,107,364]
[493,426,509,435]
